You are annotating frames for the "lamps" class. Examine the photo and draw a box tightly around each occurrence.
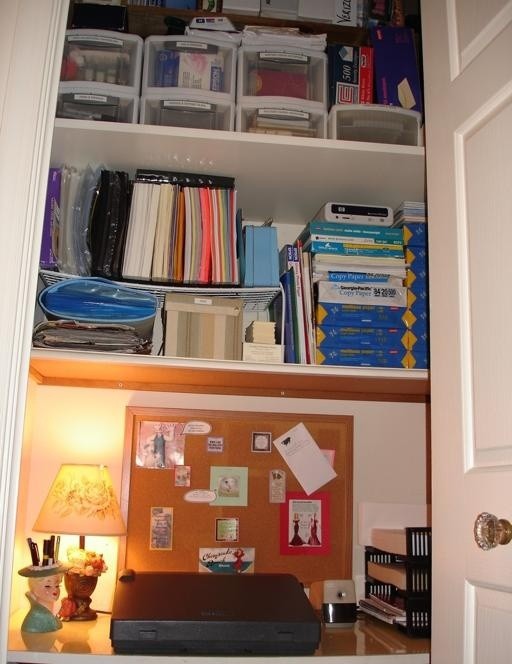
[30,462,129,623]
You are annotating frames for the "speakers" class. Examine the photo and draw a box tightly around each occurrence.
[309,579,357,628]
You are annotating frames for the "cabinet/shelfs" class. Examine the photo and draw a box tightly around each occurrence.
[362,524,430,640]
[8,0,434,661]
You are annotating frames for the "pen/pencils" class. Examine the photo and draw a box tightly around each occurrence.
[28,535,60,565]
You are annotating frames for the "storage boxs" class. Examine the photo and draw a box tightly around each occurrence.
[236,44,327,139]
[328,105,422,147]
[140,36,239,133]
[55,30,143,124]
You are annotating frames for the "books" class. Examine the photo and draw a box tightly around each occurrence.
[121,178,237,285]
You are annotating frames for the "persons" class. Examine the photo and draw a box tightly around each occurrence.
[15,565,64,633]
